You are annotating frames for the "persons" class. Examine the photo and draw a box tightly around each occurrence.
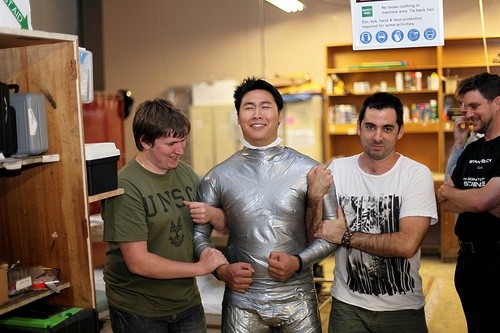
[101,97,230,333]
[304,92,439,333]
[193,78,342,333]
[435,72,500,333]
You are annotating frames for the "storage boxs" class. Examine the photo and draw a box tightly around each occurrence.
[0,0,33,29]
[84,142,120,196]
[0,305,101,333]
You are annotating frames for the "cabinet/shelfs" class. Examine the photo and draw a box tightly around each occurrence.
[0,27,126,333]
[189,96,324,180]
[326,36,500,263]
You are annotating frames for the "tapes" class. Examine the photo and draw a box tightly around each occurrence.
[32,280,45,289]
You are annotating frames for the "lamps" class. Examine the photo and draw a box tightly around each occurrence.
[265,0,308,14]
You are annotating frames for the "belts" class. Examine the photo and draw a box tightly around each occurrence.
[458,239,499,254]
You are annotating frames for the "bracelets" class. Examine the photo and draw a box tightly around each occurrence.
[293,254,302,273]
[341,226,360,246]
